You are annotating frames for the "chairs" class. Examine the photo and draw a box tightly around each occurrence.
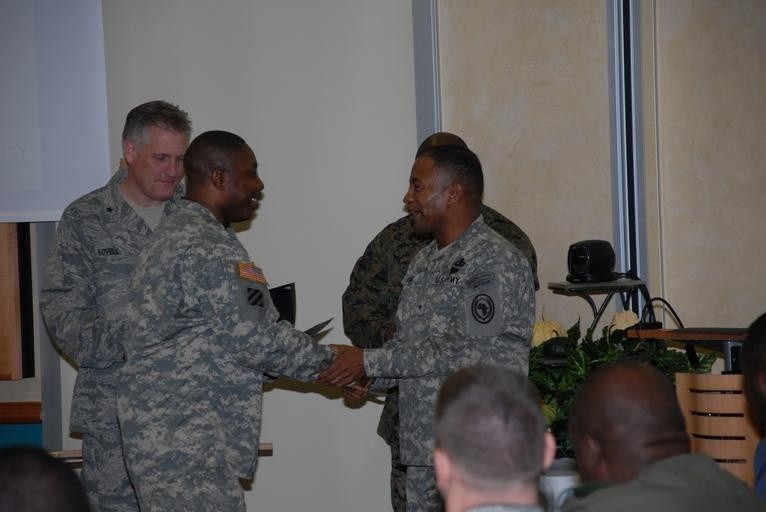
[674,373,761,489]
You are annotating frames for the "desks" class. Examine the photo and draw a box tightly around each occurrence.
[548,277,651,334]
[627,328,750,371]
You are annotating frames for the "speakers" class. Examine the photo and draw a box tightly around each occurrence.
[566,239,619,284]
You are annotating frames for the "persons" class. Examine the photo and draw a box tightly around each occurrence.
[0,444,90,512]
[558,361,766,511]
[116,130,340,512]
[317,144,536,512]
[37,100,191,512]
[739,312,766,499]
[432,364,557,512]
[342,132,539,512]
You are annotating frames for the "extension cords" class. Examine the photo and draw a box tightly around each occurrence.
[634,322,662,330]
